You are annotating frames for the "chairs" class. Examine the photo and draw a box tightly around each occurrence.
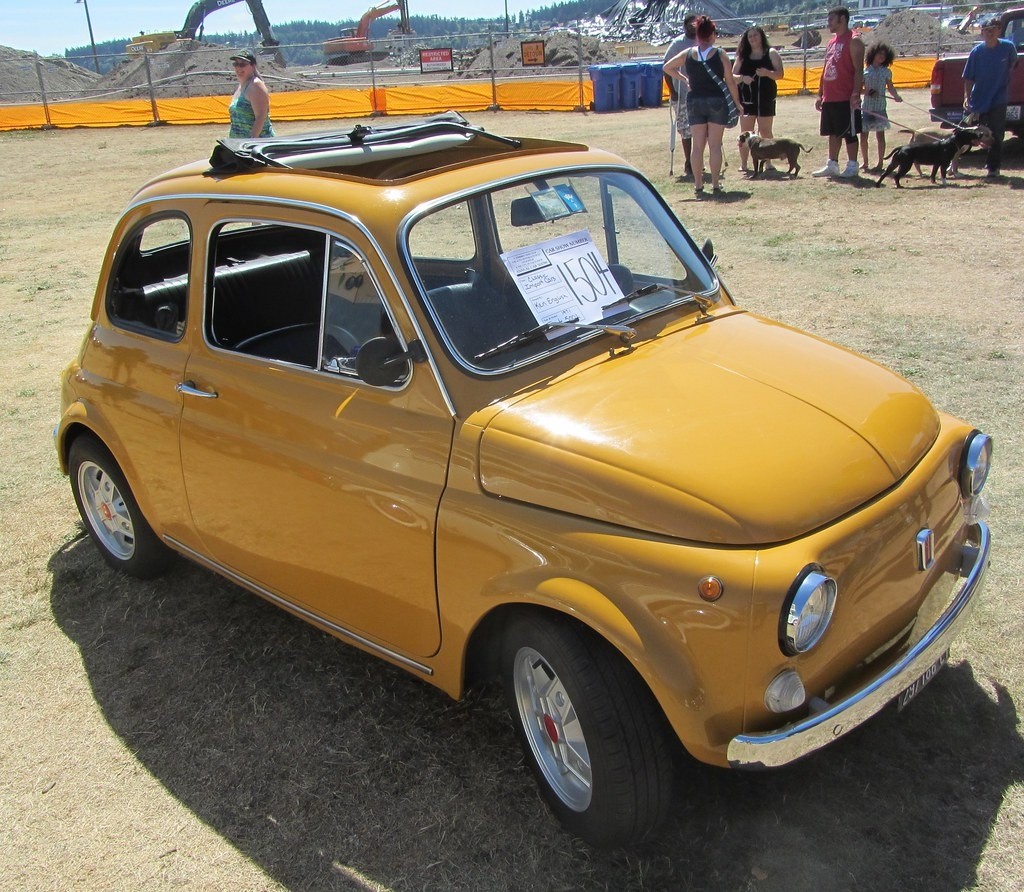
[232,322,359,371]
[429,283,482,362]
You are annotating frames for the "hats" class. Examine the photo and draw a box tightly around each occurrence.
[981,18,1000,29]
[230,49,256,63]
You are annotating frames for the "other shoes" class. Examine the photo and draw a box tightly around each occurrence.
[711,188,726,196]
[694,186,704,200]
[684,164,705,175]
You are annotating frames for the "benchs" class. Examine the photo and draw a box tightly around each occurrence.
[125,251,314,351]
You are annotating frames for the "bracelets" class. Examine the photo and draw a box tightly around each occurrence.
[739,75,743,82]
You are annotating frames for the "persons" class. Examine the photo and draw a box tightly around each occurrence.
[731,25,784,171]
[812,6,865,177]
[662,16,743,196]
[938,17,1018,177]
[228,50,276,139]
[859,40,902,171]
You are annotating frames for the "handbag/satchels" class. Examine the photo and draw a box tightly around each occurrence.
[724,84,739,128]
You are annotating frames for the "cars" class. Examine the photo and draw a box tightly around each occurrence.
[50,115,994,847]
[941,13,1001,31]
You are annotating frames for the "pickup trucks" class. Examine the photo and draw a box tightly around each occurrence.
[927,7,1024,143]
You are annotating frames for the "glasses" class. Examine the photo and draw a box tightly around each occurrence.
[232,62,249,67]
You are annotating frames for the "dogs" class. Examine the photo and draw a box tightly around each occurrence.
[736,128,815,180]
[874,122,996,189]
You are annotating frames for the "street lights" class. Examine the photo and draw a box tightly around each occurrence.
[76,0,99,74]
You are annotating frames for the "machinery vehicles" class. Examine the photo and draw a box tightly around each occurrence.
[125,0,287,68]
[322,0,413,66]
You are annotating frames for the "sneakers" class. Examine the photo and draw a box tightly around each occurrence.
[987,165,1000,176]
[841,161,859,177]
[812,159,840,177]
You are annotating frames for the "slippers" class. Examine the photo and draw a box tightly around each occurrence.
[738,163,748,171]
[765,165,776,170]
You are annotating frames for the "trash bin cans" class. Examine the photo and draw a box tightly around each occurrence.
[616,62,640,109]
[586,64,621,112]
[640,61,665,108]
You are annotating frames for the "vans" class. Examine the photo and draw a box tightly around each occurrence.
[848,15,866,30]
